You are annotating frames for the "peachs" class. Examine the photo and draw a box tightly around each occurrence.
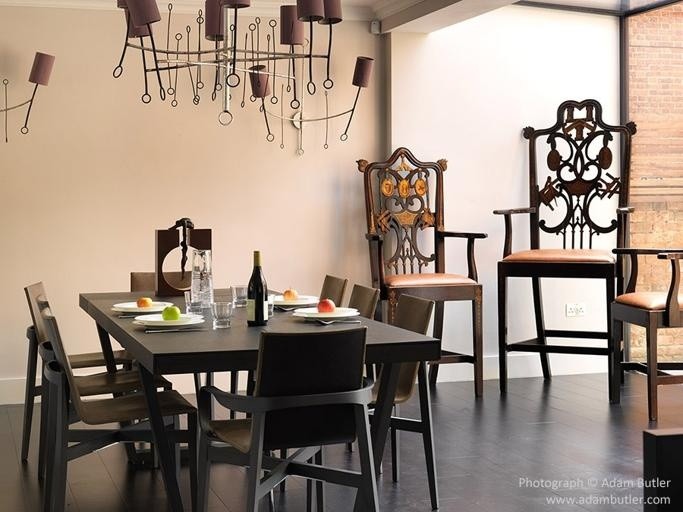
[284,290,297,301]
[137,298,153,308]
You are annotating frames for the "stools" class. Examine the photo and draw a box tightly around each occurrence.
[606,241,681,424]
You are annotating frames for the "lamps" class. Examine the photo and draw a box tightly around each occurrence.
[241,54,375,157]
[0,47,59,144]
[111,0,341,130]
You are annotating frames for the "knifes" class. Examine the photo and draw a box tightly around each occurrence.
[145,329,211,333]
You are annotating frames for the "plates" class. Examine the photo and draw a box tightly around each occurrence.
[268,294,359,321]
[110,302,206,329]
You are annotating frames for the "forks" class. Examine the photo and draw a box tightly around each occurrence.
[315,319,361,325]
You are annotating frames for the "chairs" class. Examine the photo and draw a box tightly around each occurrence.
[363,144,482,396]
[488,90,634,405]
[21,265,445,512]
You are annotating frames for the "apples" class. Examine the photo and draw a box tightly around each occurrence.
[318,298,336,312]
[162,306,181,321]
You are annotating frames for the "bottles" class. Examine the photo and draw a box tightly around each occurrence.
[191,250,214,309]
[246,251,268,327]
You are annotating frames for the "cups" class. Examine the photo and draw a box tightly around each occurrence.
[184,292,203,315]
[230,285,246,307]
[209,302,233,329]
